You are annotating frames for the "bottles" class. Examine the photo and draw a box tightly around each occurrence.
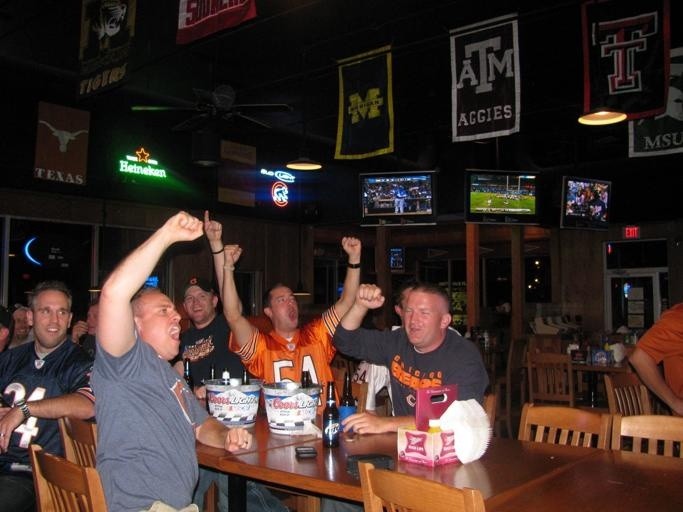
[240,369,250,386]
[321,382,341,449]
[183,357,193,391]
[427,418,444,434]
[469,325,490,351]
[338,371,356,432]
[301,370,309,387]
[220,367,232,385]
[203,365,215,412]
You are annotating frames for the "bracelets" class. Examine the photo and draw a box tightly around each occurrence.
[222,265,234,271]
[87,210,293,512]
[208,246,223,255]
[346,261,361,269]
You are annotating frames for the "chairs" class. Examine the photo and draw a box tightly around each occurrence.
[25,439,106,512]
[268,379,368,512]
[355,461,486,512]
[58,416,98,466]
[486,330,669,442]
[612,411,682,458]
[517,402,614,448]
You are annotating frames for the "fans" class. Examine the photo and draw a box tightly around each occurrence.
[130,48,290,132]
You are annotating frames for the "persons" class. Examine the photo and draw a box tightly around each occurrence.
[0,277,95,512]
[70,299,101,361]
[168,244,254,384]
[564,180,609,222]
[625,302,683,419]
[7,302,36,350]
[0,305,12,354]
[363,175,432,214]
[318,281,490,511]
[350,279,463,416]
[220,235,363,386]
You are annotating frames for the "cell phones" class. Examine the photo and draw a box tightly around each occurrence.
[296,446,317,458]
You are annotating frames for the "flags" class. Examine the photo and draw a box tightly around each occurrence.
[624,43,682,160]
[578,0,672,123]
[447,10,522,145]
[73,0,137,98]
[172,1,259,48]
[331,43,396,165]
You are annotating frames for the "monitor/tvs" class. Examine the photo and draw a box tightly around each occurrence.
[358,170,439,228]
[462,169,541,226]
[559,175,612,232]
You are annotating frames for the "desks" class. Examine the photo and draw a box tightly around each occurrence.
[192,416,682,512]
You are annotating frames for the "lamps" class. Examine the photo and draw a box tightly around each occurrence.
[291,175,310,297]
[285,0,322,170]
[576,0,627,126]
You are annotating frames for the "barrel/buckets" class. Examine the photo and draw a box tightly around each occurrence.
[201,378,262,430]
[600,333,623,364]
[260,381,324,437]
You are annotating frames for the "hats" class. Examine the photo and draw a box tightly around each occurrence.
[184,275,216,294]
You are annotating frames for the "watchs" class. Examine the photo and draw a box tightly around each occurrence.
[12,398,32,419]
